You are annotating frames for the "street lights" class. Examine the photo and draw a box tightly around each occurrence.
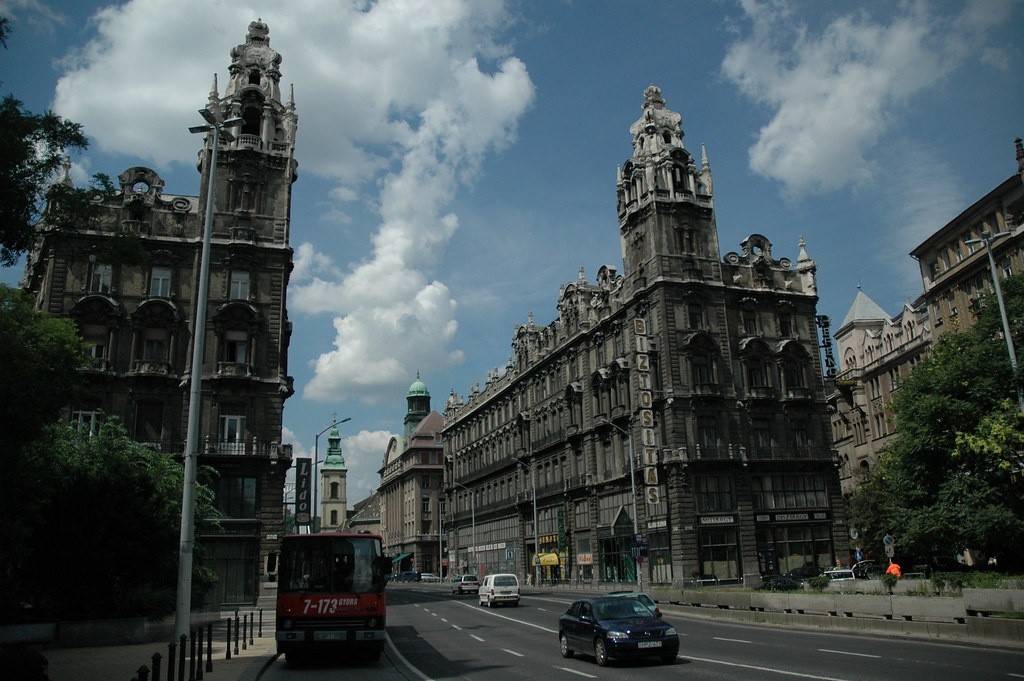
[510,457,542,587]
[172,104,246,638]
[423,495,444,583]
[600,419,643,591]
[312,417,354,533]
[454,482,477,575]
[964,229,1024,417]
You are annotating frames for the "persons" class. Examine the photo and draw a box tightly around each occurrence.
[886,561,902,579]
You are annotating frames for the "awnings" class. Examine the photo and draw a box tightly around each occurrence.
[392,552,412,563]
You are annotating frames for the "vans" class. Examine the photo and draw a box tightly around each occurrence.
[449,576,480,594]
[478,573,521,608]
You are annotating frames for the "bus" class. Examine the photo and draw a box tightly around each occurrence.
[265,529,394,666]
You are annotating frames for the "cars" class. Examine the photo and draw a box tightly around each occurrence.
[818,558,876,583]
[602,589,663,618]
[393,571,440,582]
[558,597,681,667]
[759,575,804,590]
